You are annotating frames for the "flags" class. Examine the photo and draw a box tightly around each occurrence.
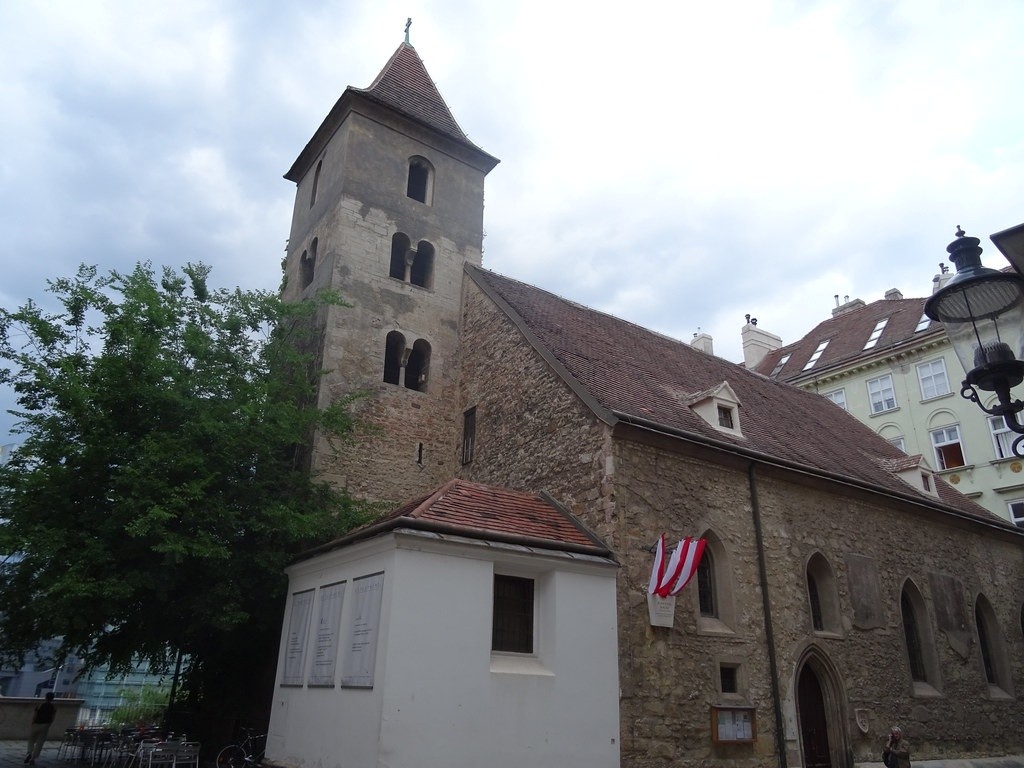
[658,536,692,597]
[648,534,666,594]
[669,539,707,597]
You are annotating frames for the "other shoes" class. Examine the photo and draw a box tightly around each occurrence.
[29,759,35,765]
[24,754,31,763]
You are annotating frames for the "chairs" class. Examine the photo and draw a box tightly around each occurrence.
[55,730,201,768]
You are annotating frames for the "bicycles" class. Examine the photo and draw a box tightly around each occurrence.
[216,727,268,768]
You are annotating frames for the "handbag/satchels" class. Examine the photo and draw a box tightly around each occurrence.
[882,751,898,768]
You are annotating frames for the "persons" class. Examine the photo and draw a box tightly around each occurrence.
[23,692,57,767]
[880,727,911,768]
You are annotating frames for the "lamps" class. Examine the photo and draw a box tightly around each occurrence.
[926,223,1024,456]
